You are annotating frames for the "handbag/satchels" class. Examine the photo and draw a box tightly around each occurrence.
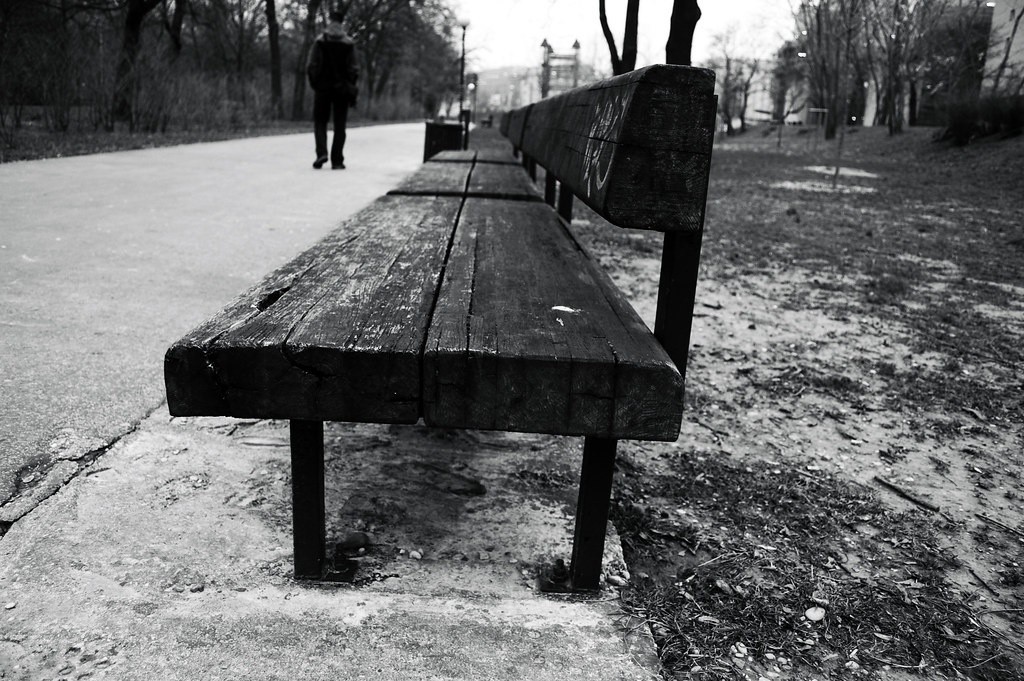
[347,84,358,108]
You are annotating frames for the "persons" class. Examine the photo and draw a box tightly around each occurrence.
[422,93,435,120]
[307,11,355,169]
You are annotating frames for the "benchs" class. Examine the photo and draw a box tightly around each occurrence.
[164,63,719,593]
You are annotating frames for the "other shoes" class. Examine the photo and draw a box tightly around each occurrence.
[313,155,328,169]
[332,164,345,169]
